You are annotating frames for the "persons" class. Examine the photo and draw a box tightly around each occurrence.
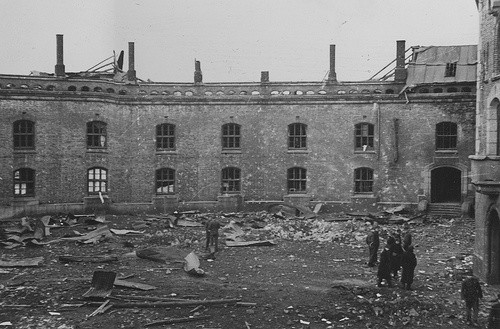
[387,236,404,276]
[209,216,220,252]
[377,245,393,287]
[400,247,418,290]
[206,217,213,251]
[403,227,412,251]
[365,225,380,267]
[395,228,402,247]
[460,269,483,327]
